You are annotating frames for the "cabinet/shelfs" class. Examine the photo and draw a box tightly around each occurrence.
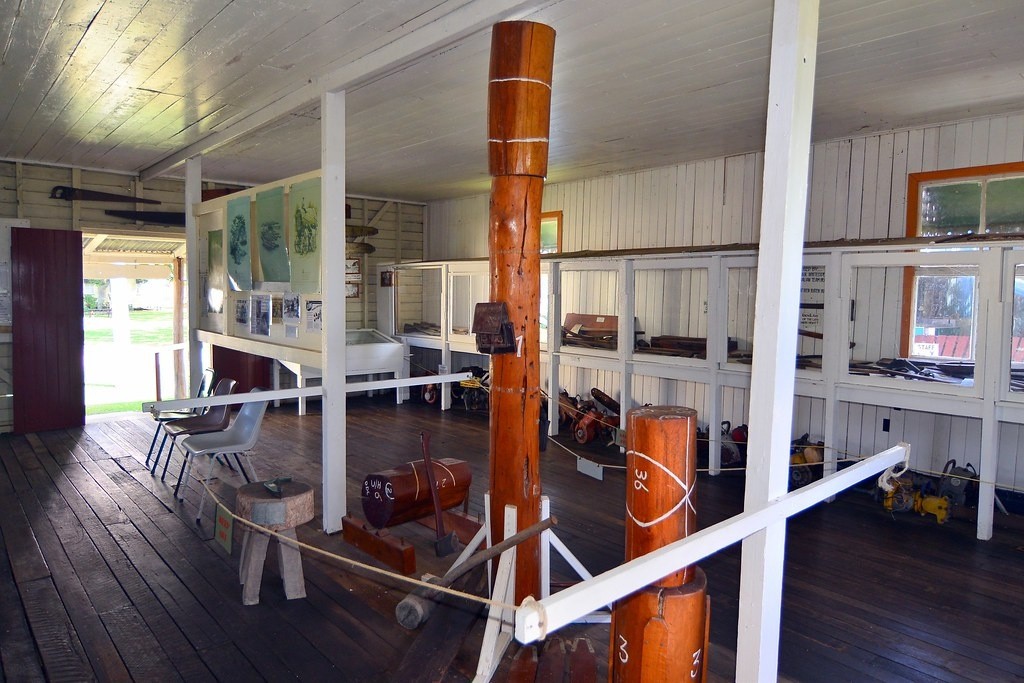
[377,250,1024,540]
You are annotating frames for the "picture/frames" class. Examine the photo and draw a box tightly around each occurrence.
[345,283,359,299]
[345,257,361,274]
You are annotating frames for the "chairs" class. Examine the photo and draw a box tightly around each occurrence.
[144,367,217,475]
[179,387,270,525]
[161,377,239,497]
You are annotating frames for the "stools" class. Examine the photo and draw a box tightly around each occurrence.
[235,477,316,605]
